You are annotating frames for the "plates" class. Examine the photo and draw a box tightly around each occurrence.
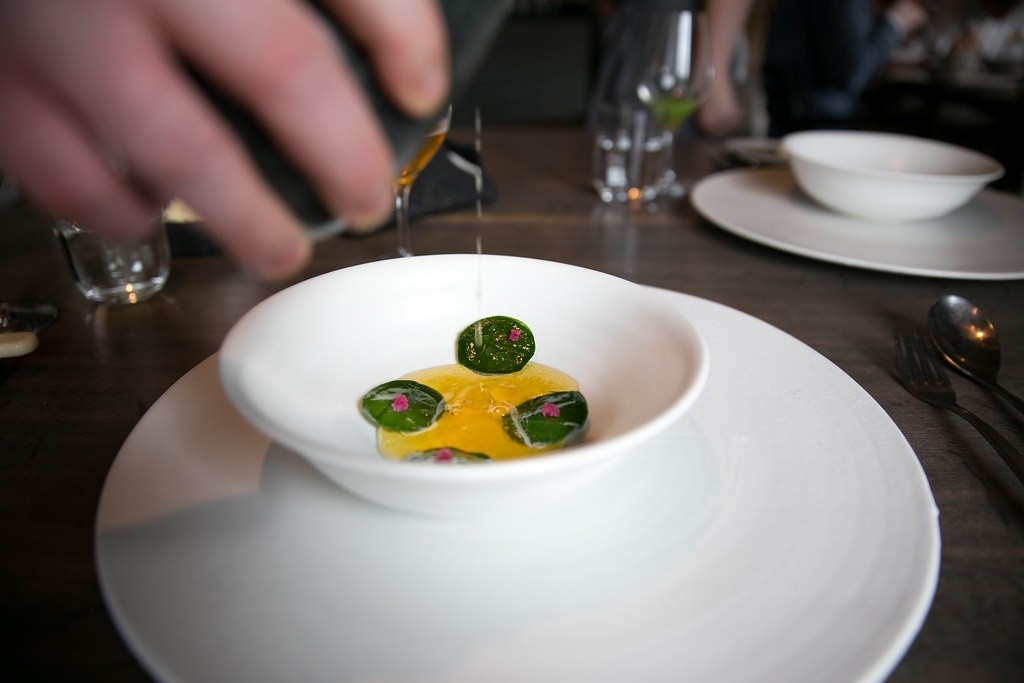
[724,138,783,163]
[97,286,941,683]
[692,170,1024,281]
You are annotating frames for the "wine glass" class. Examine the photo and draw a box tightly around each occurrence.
[635,10,715,221]
[378,105,453,260]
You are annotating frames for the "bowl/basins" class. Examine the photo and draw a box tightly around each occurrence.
[219,254,710,514]
[785,129,1003,219]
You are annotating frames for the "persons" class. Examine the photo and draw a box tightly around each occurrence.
[1,0,1024,285]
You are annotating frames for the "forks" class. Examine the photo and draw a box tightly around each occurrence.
[894,326,1024,485]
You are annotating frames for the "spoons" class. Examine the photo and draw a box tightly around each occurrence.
[930,294,1024,417]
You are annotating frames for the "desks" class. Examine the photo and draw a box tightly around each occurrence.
[884,61,1024,153]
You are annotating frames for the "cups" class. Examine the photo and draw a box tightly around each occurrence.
[52,196,173,303]
[581,51,673,202]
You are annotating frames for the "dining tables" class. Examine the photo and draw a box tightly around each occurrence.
[0,125,1024,683]
